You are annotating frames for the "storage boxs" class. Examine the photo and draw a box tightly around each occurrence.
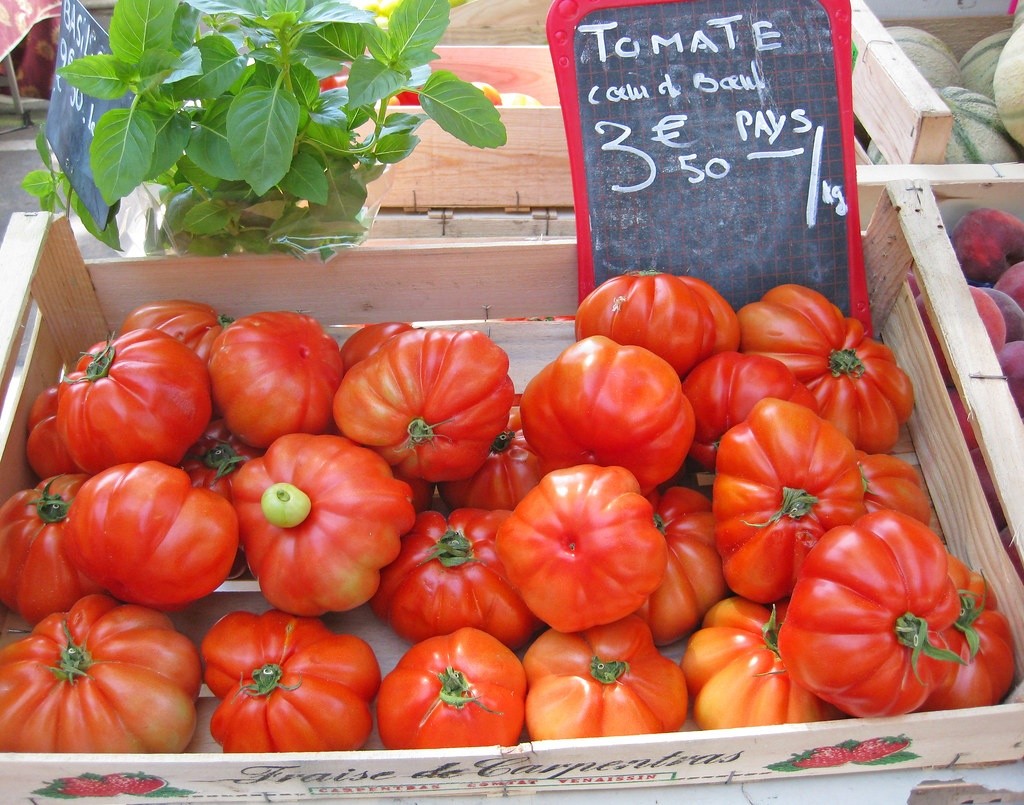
[0,1,1024,805]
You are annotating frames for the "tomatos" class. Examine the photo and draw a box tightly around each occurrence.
[0,270,1017,753]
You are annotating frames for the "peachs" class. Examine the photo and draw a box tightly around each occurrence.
[901,208,1024,589]
